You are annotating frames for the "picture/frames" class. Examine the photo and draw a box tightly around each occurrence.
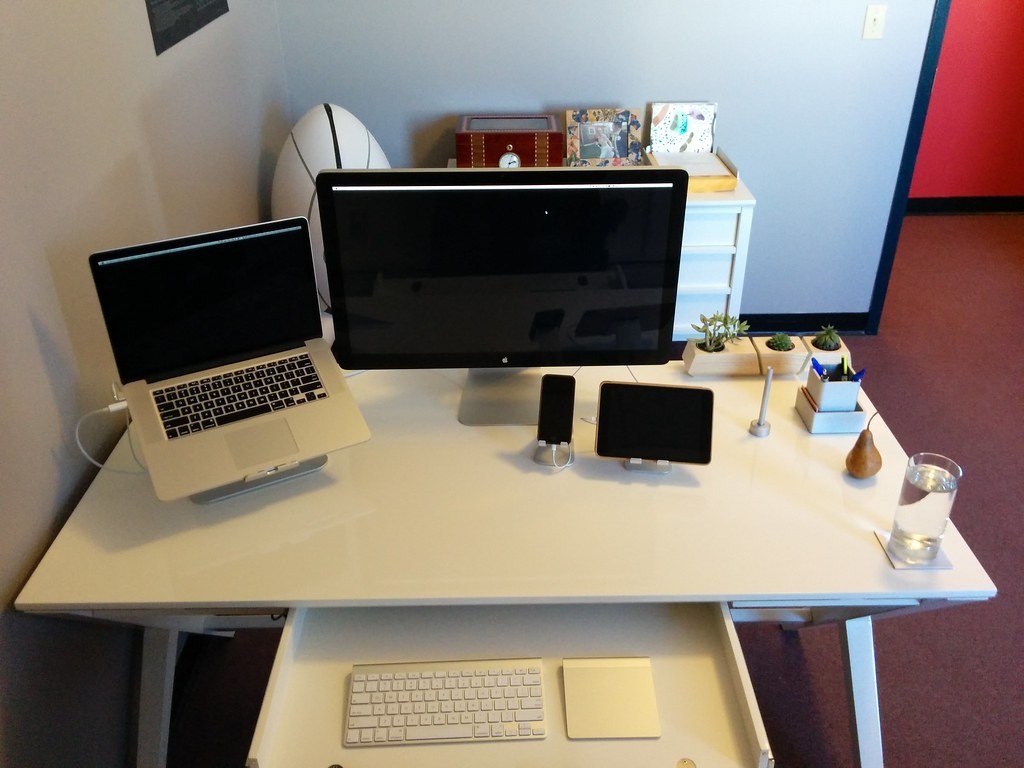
[563,106,643,170]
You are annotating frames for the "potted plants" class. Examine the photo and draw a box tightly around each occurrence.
[681,310,852,377]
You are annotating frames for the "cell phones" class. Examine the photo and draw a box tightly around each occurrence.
[537,374,576,445]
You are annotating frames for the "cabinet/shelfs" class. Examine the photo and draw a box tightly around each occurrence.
[444,158,758,343]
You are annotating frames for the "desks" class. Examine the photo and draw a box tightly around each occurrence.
[11,333,996,767]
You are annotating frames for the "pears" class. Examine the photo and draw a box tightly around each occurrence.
[846,411,882,479]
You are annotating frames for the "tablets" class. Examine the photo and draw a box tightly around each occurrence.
[595,381,713,465]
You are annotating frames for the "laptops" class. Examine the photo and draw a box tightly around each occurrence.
[88,216,371,498]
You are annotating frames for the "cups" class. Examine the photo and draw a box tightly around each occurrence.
[886,452,964,566]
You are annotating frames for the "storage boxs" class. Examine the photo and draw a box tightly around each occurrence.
[453,111,565,168]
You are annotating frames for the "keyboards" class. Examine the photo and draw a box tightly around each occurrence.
[344,655,546,750]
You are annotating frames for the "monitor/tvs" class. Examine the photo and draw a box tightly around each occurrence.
[315,165,688,424]
